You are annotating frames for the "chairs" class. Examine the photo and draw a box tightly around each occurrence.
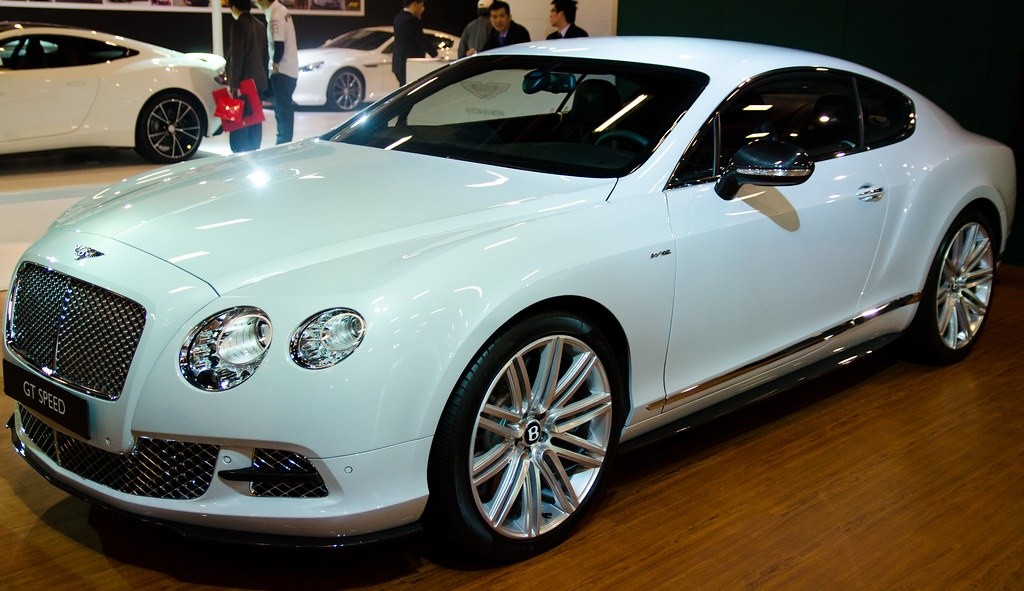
[559,78,620,149]
[23,40,46,69]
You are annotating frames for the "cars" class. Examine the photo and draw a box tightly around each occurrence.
[0,38,58,60]
[291,25,460,112]
[1,28,228,164]
[1,32,1016,560]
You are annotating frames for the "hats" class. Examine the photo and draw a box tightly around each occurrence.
[477,0,493,8]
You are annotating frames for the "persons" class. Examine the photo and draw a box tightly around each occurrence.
[392,0,445,127]
[545,0,589,40]
[458,0,530,60]
[253,0,298,145]
[224,0,270,152]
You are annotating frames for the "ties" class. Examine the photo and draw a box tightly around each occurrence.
[558,32,562,38]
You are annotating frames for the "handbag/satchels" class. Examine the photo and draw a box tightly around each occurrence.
[212,78,265,132]
